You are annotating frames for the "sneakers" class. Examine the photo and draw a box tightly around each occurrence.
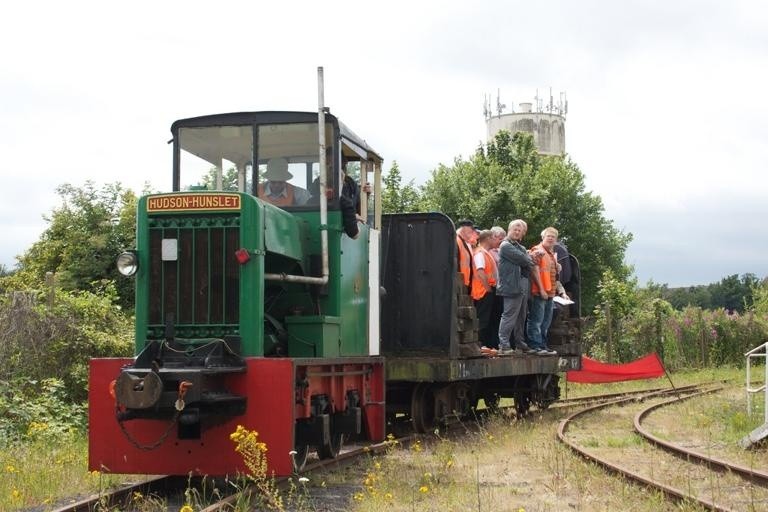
[481,343,557,355]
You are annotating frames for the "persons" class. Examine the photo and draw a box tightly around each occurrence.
[456,219,570,355]
[310,146,371,216]
[305,167,366,240]
[258,158,306,206]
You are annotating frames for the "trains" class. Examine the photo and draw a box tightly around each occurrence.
[88,65,583,479]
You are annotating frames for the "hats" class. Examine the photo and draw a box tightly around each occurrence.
[262,159,293,180]
[458,220,479,230]
